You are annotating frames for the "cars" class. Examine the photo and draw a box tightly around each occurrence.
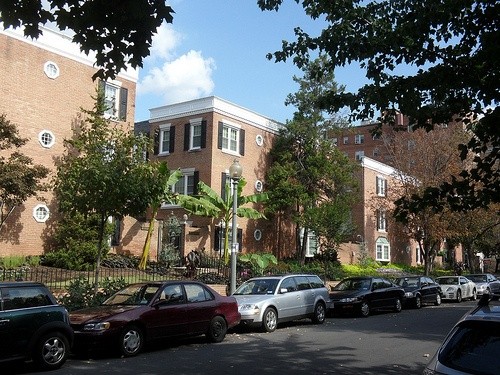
[468,274,500,302]
[435,276,478,303]
[69,279,241,357]
[231,273,330,332]
[422,295,500,375]
[0,282,74,371]
[393,275,443,309]
[328,276,405,317]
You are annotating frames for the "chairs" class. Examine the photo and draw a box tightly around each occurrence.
[144,289,208,305]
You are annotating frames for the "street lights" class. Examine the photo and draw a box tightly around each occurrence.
[228,158,243,296]
